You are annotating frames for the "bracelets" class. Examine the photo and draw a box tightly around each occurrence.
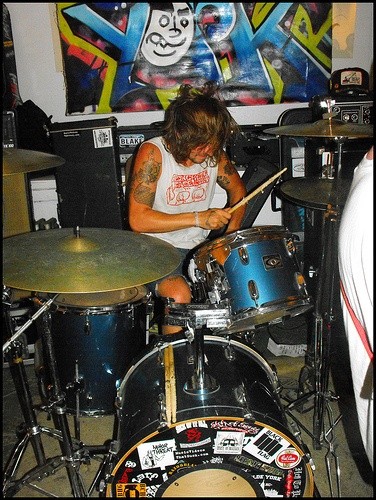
[194,211,200,228]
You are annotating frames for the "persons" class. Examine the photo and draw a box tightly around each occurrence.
[128,82,248,338]
[337,90,376,470]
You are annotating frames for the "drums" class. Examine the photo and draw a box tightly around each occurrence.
[34,285,152,418]
[190,225,314,335]
[102,336,314,499]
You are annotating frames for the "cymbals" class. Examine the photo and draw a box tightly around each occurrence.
[263,120,374,137]
[3,228,181,293]
[2,148,65,176]
[275,177,354,212]
